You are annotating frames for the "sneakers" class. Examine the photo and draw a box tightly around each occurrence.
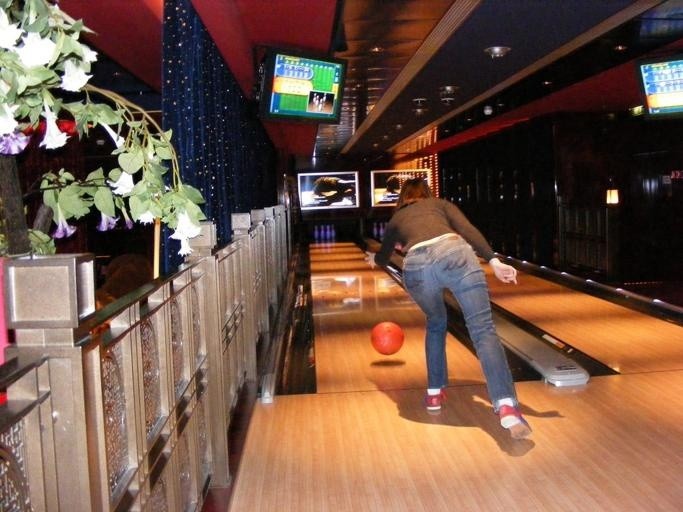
[497,403,532,441]
[423,387,449,412]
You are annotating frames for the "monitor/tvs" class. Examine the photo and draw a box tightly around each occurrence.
[636,54,683,119]
[263,48,348,124]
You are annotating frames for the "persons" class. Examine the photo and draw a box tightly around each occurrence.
[360,179,532,442]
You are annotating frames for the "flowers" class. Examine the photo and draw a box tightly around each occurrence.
[0,3,205,241]
